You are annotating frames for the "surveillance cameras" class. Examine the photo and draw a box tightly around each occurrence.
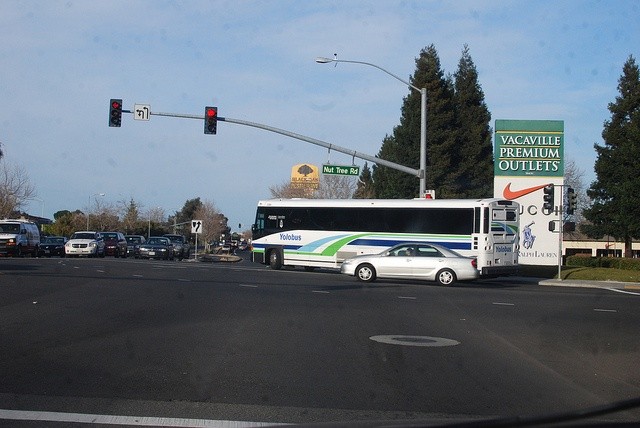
[333,52,338,62]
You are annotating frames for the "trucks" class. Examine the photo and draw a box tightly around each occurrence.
[0,219,40,257]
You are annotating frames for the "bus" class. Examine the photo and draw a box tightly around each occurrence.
[250,198,520,276]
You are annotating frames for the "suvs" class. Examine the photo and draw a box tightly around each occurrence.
[162,234,189,261]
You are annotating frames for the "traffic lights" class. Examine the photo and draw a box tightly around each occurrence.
[205,106,217,135]
[543,184,554,211]
[567,187,577,214]
[109,99,122,127]
[424,193,432,199]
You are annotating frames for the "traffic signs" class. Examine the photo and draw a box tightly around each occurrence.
[323,165,359,175]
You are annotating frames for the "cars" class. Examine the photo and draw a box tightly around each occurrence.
[340,243,481,287]
[64,231,105,257]
[221,244,231,252]
[124,235,145,258]
[39,237,67,258]
[100,232,128,258]
[139,237,174,260]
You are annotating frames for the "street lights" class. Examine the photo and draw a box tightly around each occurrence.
[316,57,427,198]
[87,193,105,232]
